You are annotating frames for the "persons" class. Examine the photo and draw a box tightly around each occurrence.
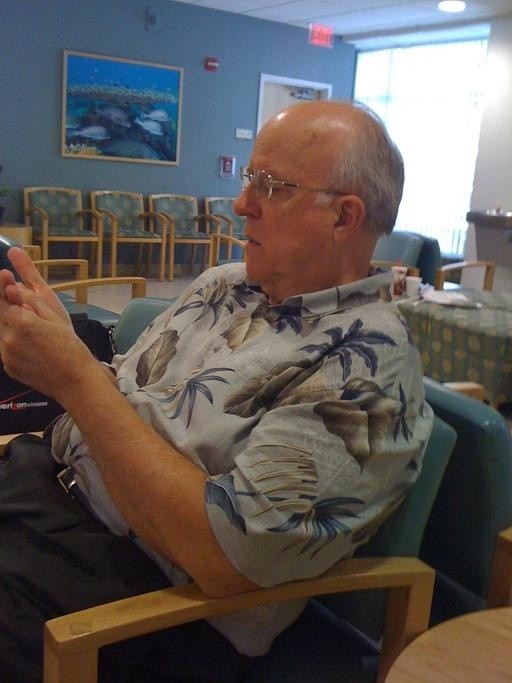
[1,100,436,682]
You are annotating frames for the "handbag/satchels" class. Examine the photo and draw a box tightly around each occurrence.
[0,313,117,435]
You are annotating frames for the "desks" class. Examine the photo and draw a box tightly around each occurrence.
[396,284,512,410]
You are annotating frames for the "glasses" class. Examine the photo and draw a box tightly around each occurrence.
[239,166,352,200]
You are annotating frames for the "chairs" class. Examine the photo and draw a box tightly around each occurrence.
[370,229,496,291]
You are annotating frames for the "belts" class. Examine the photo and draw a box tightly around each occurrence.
[55,464,109,527]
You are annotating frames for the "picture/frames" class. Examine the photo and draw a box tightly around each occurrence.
[61,48,185,167]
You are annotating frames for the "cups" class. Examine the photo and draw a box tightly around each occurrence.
[406,275,422,295]
[392,265,408,295]
